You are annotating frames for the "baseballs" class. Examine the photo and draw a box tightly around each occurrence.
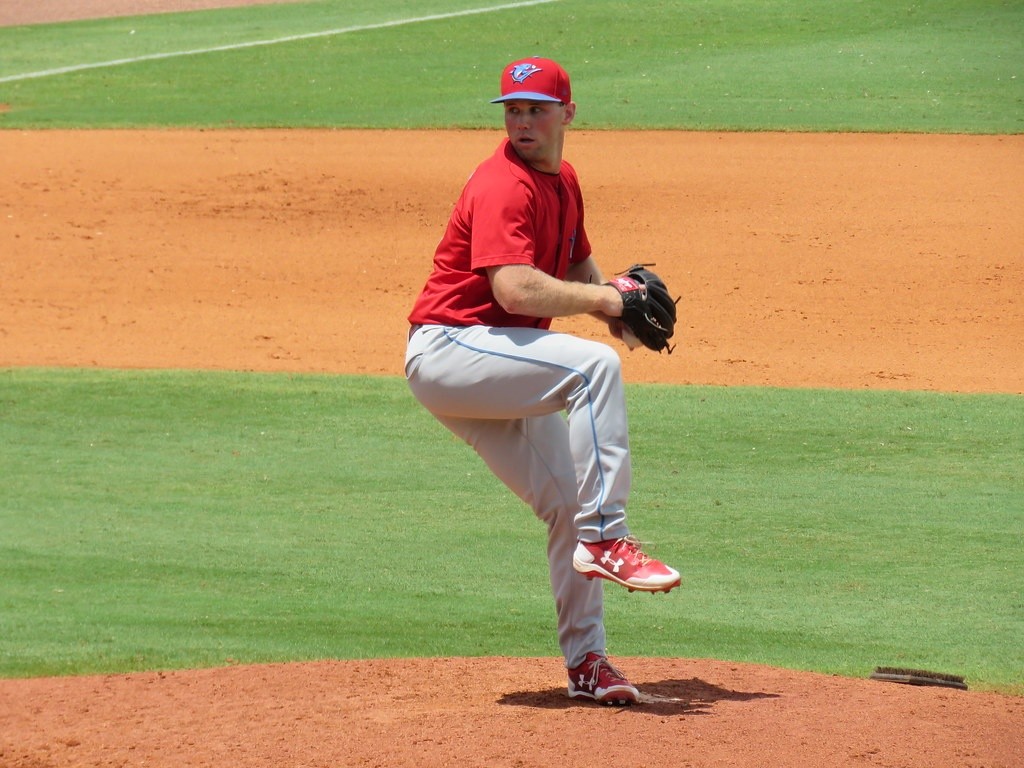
[620,323,644,350]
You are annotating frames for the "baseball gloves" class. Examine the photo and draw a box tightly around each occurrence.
[609,265,677,355]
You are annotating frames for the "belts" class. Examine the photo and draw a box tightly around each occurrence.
[409,322,422,340]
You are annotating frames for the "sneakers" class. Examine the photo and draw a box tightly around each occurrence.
[567,652,639,705]
[573,537,682,595]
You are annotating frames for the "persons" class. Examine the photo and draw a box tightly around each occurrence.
[403,56,684,706]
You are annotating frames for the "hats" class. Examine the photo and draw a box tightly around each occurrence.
[490,56,571,105]
